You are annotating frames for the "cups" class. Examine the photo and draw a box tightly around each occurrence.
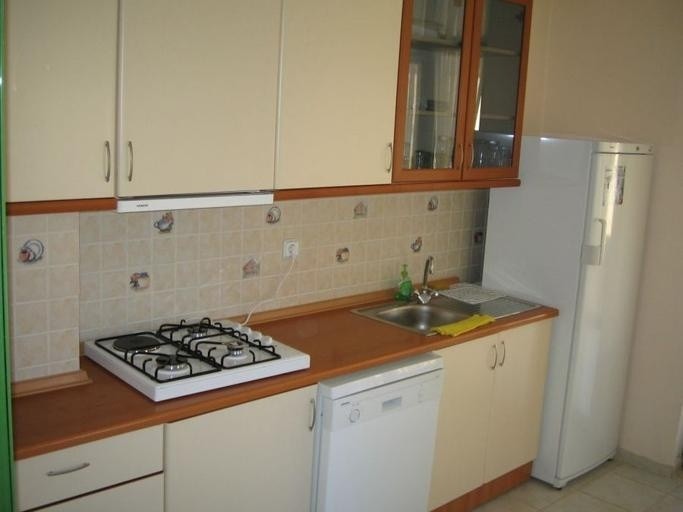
[405,133,509,169]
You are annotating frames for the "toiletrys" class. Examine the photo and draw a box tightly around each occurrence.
[394,264,415,300]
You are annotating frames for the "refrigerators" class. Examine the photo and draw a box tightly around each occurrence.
[481,134,654,490]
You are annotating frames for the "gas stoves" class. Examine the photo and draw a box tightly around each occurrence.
[82,312,311,404]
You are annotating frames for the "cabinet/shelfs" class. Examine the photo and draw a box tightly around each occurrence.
[276,2,405,203]
[429,318,556,512]
[399,0,534,194]
[162,384,314,509]
[14,418,167,510]
[1,2,280,216]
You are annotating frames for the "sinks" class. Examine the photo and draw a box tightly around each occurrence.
[351,298,476,338]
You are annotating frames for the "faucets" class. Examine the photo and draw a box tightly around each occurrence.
[422,256,434,292]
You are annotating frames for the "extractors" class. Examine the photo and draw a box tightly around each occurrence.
[115,192,274,214]
